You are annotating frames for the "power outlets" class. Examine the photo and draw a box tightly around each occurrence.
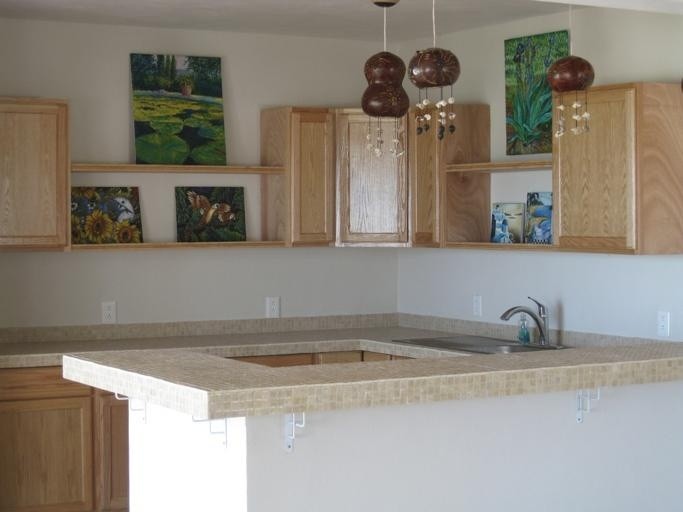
[473,296,481,317]
[658,312,670,338]
[266,296,280,319]
[100,301,117,324]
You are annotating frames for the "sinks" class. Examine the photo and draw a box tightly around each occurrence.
[392,329,562,355]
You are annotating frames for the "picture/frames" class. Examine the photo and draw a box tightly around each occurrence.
[70,165,288,249]
[333,108,439,247]
[1,99,70,248]
[553,81,683,256]
[69,184,147,245]
[173,184,250,244]
[436,103,554,251]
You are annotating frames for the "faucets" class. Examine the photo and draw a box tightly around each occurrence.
[500,295,550,347]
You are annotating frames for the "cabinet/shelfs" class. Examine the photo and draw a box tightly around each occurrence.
[94,386,129,512]
[0,367,94,512]
[227,349,419,366]
[258,104,332,245]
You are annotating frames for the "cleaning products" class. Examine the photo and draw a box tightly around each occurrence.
[518,313,531,341]
